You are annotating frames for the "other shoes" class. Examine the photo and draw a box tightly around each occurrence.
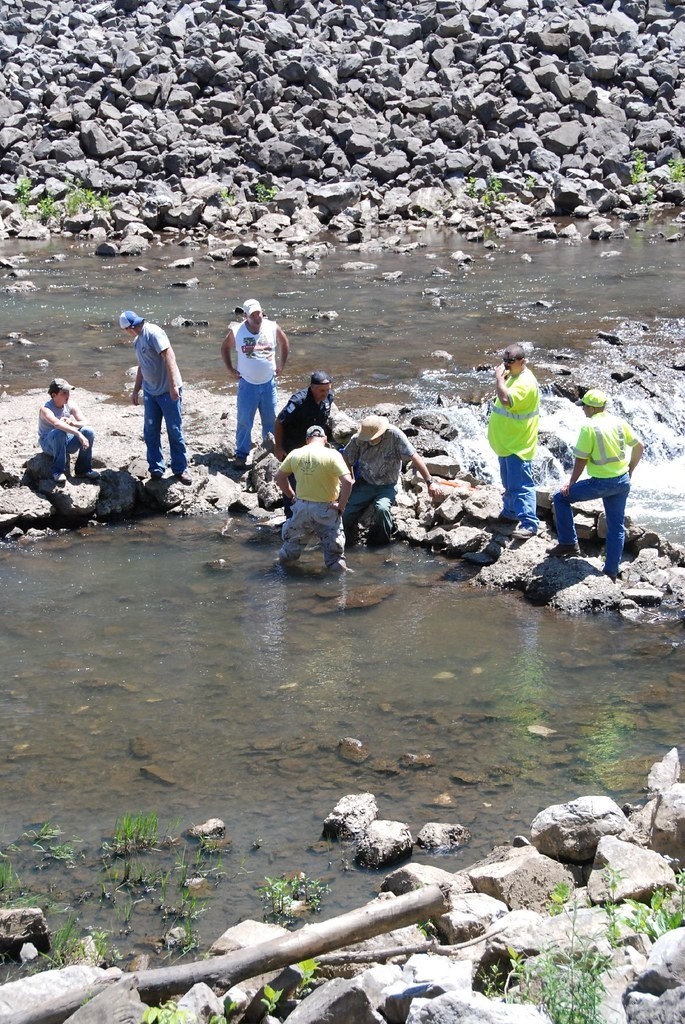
[151,472,163,480]
[176,471,193,484]
[235,456,246,468]
[545,542,581,555]
[53,472,66,482]
[487,513,519,524]
[512,527,533,538]
[76,469,101,479]
[602,569,616,584]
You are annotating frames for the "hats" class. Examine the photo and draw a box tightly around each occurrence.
[311,371,331,385]
[357,413,389,441]
[243,299,261,316]
[119,310,146,329]
[306,425,325,437]
[582,389,607,408]
[50,378,75,391]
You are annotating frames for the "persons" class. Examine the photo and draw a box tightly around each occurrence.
[546,390,644,582]
[119,311,191,483]
[221,299,290,469]
[275,373,334,521]
[275,426,352,573]
[344,414,443,549]
[38,378,100,482]
[488,345,538,538]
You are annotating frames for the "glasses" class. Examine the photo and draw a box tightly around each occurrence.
[502,357,523,364]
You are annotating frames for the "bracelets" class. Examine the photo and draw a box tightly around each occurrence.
[426,479,432,484]
